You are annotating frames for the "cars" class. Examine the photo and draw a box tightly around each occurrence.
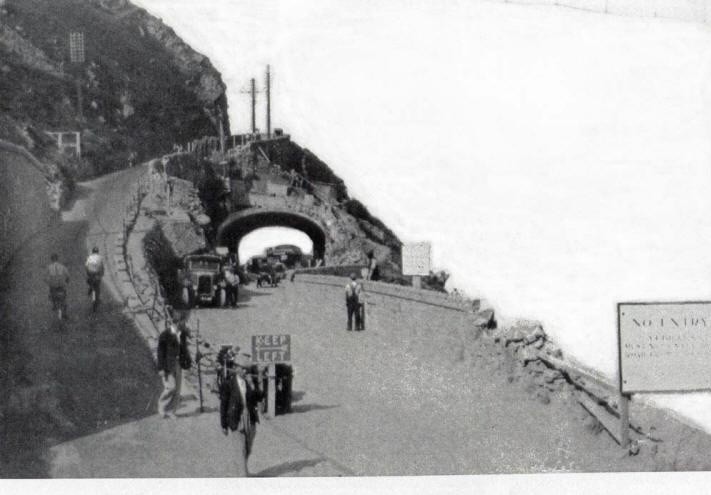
[180,254,232,309]
[246,244,302,280]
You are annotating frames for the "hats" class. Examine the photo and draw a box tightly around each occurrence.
[232,352,252,370]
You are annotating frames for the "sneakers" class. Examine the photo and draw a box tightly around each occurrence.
[158,407,179,420]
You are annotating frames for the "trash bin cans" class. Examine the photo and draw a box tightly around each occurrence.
[264,363,292,414]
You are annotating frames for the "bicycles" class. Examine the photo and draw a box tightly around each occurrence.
[48,273,100,331]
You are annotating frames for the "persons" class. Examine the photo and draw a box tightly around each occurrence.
[222,253,239,308]
[43,253,71,312]
[158,312,191,419]
[220,360,263,477]
[344,273,365,331]
[84,247,105,301]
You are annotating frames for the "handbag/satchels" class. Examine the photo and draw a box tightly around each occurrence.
[179,334,192,370]
